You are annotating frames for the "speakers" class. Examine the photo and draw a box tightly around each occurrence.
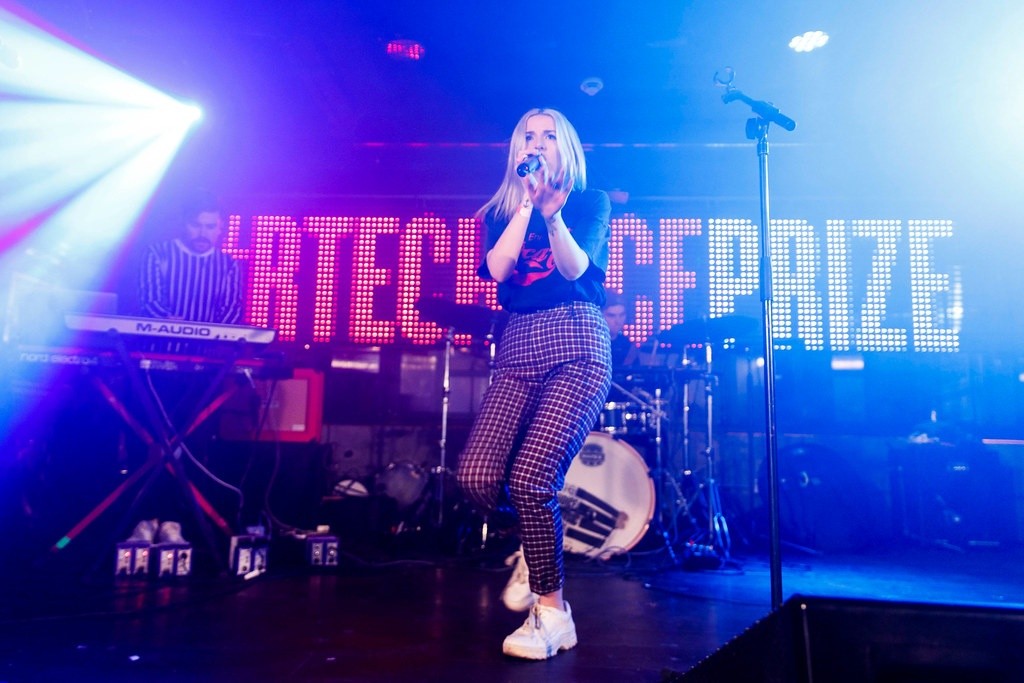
[672,592,1024,683]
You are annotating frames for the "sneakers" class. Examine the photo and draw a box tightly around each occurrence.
[502,601,577,660]
[500,541,536,611]
[127,515,187,546]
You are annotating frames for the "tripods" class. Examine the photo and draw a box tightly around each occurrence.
[417,326,456,530]
[649,340,738,571]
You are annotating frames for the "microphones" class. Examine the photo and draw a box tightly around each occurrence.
[516,155,541,177]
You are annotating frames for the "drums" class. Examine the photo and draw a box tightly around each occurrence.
[557,428,656,560]
[594,401,655,435]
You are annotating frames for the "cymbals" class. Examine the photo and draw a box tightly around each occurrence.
[657,314,761,345]
[411,298,509,336]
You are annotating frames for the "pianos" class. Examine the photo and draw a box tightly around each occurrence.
[13,311,296,382]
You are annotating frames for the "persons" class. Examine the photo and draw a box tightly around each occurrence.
[604,291,642,399]
[116,189,241,545]
[454,108,612,659]
